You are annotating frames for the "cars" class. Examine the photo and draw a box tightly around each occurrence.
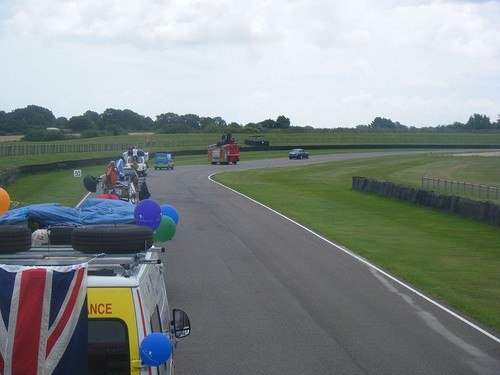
[126,156,149,176]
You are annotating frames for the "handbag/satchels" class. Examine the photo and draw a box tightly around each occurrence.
[104,174,112,184]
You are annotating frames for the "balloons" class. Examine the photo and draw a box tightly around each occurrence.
[133,199,162,231]
[139,332,172,366]
[161,204,179,225]
[0,188,10,216]
[152,215,176,242]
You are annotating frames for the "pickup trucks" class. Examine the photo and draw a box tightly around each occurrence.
[289,149,311,160]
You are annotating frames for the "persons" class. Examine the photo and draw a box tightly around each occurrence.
[217,132,237,148]
[115,159,124,181]
[104,160,121,194]
[127,147,144,158]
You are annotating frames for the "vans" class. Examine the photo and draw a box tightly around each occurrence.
[153,153,175,170]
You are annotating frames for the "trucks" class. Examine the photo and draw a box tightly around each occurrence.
[207,143,241,164]
[0,244,191,375]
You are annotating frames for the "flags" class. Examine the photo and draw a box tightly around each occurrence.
[0,262,89,375]
